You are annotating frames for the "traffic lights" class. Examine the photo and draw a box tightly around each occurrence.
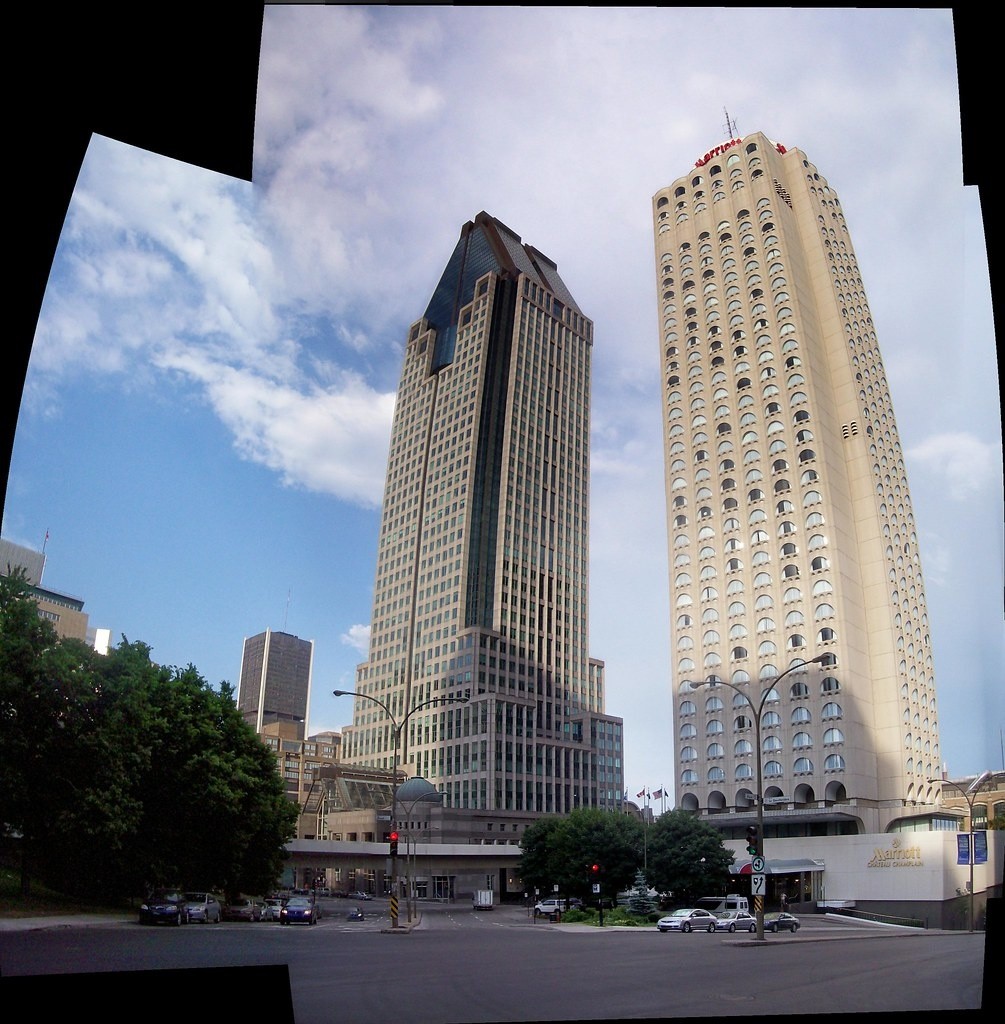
[590,864,600,875]
[746,824,757,855]
[389,831,398,857]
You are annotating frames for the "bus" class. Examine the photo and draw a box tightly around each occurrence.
[691,894,749,918]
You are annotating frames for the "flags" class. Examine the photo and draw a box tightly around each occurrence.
[623,786,669,800]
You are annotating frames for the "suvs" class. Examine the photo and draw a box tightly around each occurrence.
[534,899,574,916]
[140,887,190,926]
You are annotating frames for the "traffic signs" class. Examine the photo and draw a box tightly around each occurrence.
[745,793,758,801]
[762,795,791,805]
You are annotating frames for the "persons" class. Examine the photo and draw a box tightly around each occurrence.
[780,892,787,908]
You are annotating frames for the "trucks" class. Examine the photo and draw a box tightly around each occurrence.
[472,889,493,912]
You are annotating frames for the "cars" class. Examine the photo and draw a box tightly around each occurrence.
[764,911,801,933]
[714,910,757,933]
[657,909,718,933]
[184,892,222,924]
[222,887,368,926]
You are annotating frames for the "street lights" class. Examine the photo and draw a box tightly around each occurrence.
[928,771,1005,932]
[688,653,830,941]
[333,690,468,928]
[368,789,449,922]
